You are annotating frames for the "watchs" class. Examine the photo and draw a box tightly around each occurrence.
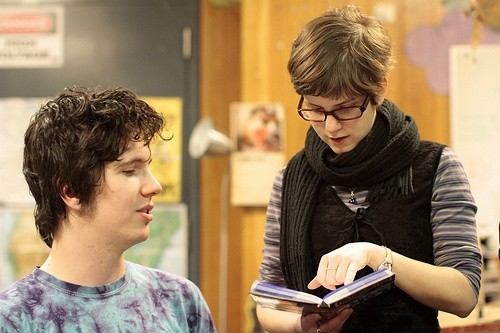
[377,245,393,271]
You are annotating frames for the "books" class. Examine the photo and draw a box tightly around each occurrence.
[249,267,396,324]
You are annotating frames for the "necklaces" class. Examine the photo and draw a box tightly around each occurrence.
[348,187,357,203]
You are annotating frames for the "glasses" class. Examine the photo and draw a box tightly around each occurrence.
[297,92,371,122]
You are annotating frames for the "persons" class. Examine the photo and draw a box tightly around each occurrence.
[0,84,218,333]
[254,4,483,333]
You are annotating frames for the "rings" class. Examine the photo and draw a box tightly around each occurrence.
[317,329,326,333]
